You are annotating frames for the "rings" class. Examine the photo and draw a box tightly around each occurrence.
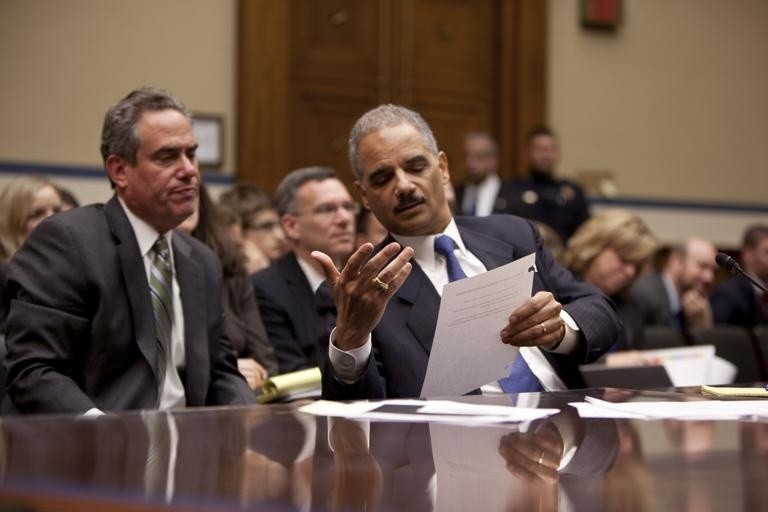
[373,277,389,291]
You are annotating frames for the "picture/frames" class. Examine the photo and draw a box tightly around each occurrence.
[191,110,225,169]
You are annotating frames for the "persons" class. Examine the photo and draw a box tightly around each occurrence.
[306,388,621,512]
[0,408,290,512]
[455,127,768,348]
[181,165,387,388]
[311,106,622,402]
[1,87,259,417]
[0,176,79,265]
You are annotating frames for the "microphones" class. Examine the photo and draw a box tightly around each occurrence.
[715,253,768,292]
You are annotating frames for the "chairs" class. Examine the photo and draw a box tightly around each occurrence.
[580,324,764,387]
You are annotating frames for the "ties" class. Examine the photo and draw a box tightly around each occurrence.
[470,186,477,215]
[147,237,172,406]
[434,235,545,393]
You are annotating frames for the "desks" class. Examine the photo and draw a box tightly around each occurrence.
[0,386,768,512]
